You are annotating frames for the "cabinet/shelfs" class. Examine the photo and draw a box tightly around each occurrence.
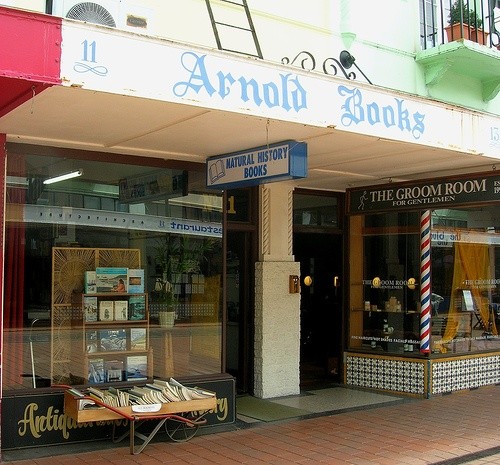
[70,293,151,385]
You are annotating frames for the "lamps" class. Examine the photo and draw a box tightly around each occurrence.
[340,50,373,85]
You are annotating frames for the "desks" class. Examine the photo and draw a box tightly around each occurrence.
[63,388,217,423]
[148,322,221,381]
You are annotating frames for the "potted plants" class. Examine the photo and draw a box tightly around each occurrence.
[443,0,488,46]
[152,235,219,328]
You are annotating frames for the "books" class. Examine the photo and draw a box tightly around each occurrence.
[66,267,214,411]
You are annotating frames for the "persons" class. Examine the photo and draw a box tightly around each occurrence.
[115,279,125,292]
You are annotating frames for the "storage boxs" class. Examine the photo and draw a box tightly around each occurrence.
[83,268,147,384]
